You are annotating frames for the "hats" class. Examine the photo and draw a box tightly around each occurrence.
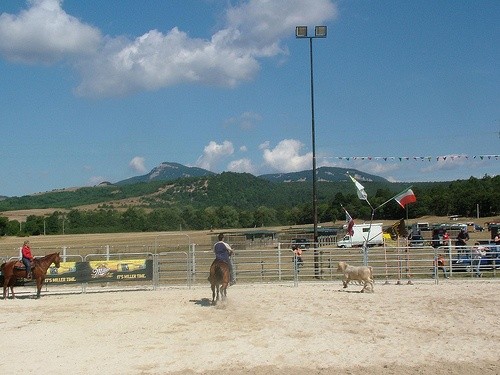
[461,229,467,234]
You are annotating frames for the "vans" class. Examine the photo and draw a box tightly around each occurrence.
[336,223,384,248]
[439,223,468,239]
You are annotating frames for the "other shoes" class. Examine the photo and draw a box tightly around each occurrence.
[460,260,463,262]
[456,261,460,263]
[480,273,483,277]
[445,276,449,278]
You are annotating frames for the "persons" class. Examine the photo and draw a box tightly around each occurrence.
[213,234,236,285]
[291,245,302,274]
[22,241,35,280]
[143,254,154,281]
[434,229,486,279]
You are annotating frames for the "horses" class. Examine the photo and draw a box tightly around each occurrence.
[210,258,230,306]
[0,252,61,299]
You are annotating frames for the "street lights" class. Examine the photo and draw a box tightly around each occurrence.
[295,25,329,280]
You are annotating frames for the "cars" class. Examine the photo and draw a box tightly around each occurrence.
[408,231,423,244]
[452,244,500,277]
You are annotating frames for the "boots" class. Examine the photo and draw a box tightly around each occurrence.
[27,271,30,279]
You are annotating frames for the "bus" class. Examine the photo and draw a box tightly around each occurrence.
[416,222,430,232]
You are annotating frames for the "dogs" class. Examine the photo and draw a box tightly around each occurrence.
[337,261,376,292]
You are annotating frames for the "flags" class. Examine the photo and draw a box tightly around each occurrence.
[345,212,354,237]
[395,189,416,209]
[349,173,367,200]
